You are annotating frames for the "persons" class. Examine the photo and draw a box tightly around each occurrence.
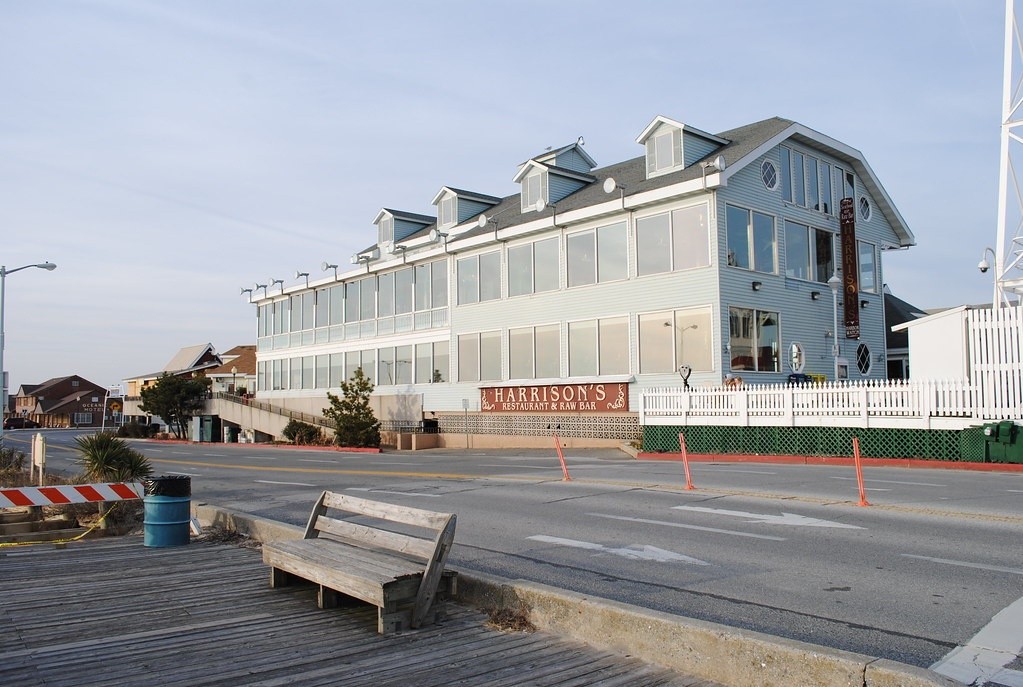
[33,423,64,428]
[734,376,746,391]
[65,424,70,428]
[724,373,736,391]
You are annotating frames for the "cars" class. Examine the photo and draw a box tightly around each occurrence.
[3,417,41,430]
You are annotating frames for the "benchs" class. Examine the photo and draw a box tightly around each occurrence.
[262,489,458,635]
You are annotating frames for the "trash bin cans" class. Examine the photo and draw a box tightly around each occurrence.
[142,475,192,547]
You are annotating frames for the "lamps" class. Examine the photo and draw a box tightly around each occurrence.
[239,156,725,316]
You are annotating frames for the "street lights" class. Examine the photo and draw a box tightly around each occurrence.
[665,320,698,366]
[978,248,998,311]
[0,261,57,447]
